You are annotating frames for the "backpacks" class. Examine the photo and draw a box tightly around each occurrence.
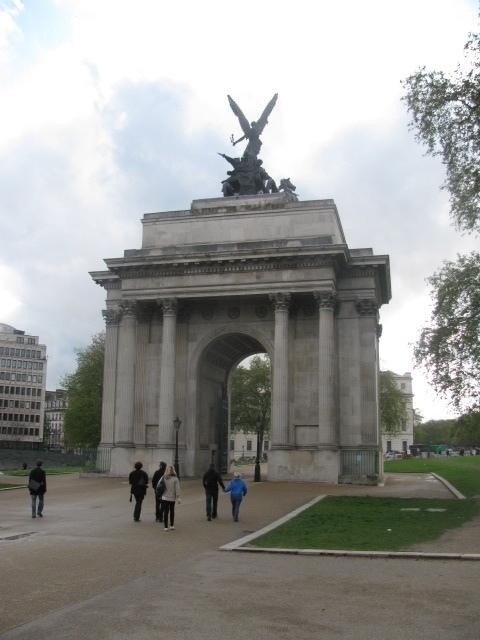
[133,470,148,496]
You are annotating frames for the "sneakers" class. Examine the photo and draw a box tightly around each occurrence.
[134,517,175,531]
[32,513,43,518]
[207,512,239,522]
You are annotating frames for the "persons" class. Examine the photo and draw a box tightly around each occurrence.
[156,464,181,530]
[127,461,149,522]
[26,460,46,518]
[151,461,167,521]
[243,121,262,156]
[224,471,247,521]
[219,156,300,202]
[201,463,226,521]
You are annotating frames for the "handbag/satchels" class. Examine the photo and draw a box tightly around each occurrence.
[156,476,167,496]
[27,480,40,494]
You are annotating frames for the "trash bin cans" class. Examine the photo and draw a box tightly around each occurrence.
[21,462,26,471]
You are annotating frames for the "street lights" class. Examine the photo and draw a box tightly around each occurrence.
[173,416,182,478]
[255,415,262,481]
